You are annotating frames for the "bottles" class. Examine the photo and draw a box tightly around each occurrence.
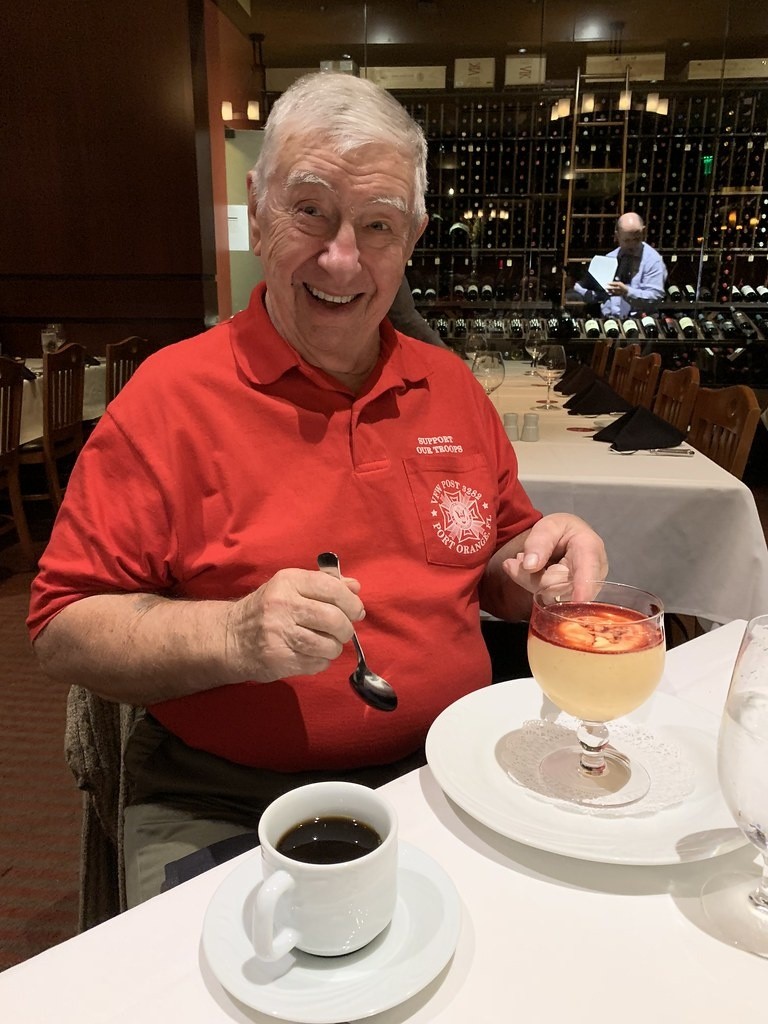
[504,413,519,441]
[405,97,768,387]
[521,413,540,442]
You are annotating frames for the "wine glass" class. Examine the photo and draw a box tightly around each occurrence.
[464,329,567,412]
[526,581,668,807]
[698,614,768,959]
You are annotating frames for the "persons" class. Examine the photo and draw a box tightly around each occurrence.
[27,73,610,909]
[573,212,668,320]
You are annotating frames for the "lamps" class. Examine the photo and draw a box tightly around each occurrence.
[550,86,669,120]
[247,100,259,120]
[222,100,233,120]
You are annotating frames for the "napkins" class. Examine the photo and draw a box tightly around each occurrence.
[554,362,688,450]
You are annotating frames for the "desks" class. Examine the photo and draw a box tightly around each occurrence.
[459,360,767,643]
[2,631,768,1024]
[0,357,132,452]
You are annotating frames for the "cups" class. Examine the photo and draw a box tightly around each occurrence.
[41,323,62,354]
[252,781,397,963]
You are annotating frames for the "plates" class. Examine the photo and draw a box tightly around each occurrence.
[202,847,461,1024]
[424,676,767,866]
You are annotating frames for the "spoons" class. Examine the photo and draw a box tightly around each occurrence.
[317,552,397,711]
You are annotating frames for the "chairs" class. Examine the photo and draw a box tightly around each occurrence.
[16,343,86,511]
[0,356,38,569]
[84,336,157,435]
[589,333,759,652]
